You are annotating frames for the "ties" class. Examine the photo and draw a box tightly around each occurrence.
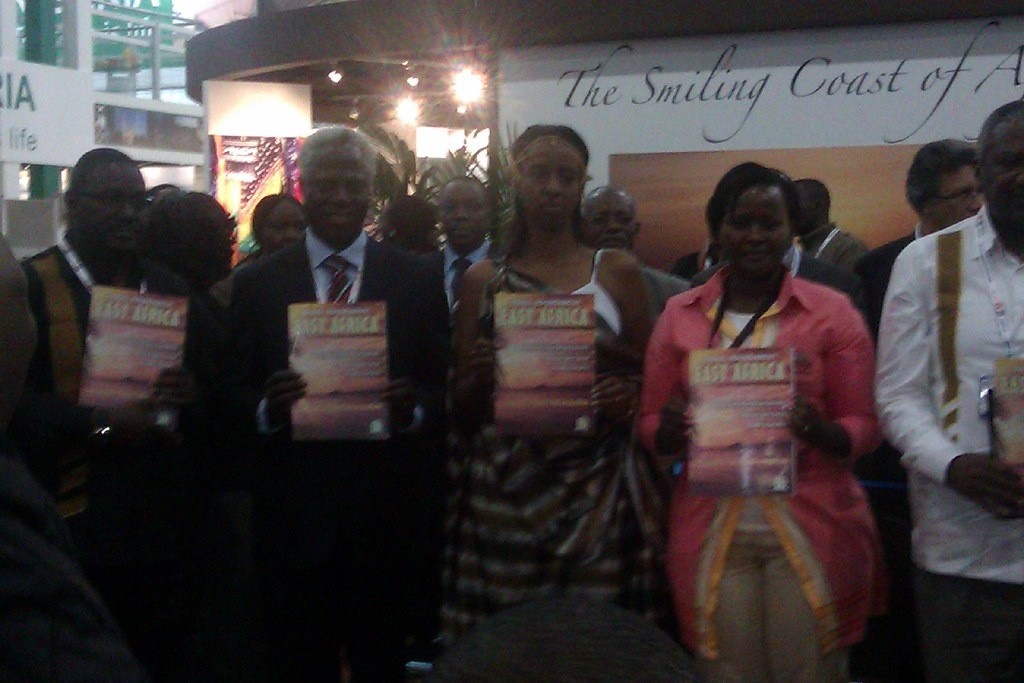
[319,255,358,304]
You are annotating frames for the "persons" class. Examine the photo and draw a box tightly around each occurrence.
[0,226,160,682]
[636,160,886,683]
[443,124,671,654]
[145,111,993,354]
[18,145,230,683]
[872,99,1024,682]
[201,124,450,682]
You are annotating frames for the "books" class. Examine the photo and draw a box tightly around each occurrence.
[281,301,393,443]
[488,291,602,442]
[682,343,798,500]
[80,282,190,415]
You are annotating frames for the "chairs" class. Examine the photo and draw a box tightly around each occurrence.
[417,600,712,683]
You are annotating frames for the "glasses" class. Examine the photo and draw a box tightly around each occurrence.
[74,186,146,209]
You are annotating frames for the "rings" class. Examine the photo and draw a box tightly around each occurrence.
[802,424,809,434]
